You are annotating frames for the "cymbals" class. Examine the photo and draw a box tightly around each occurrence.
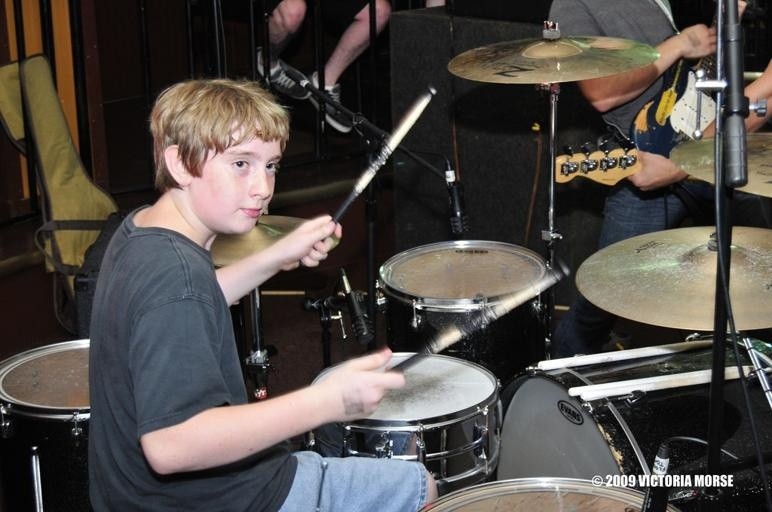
[203,215,342,271]
[448,35,659,84]
[575,224,771,332]
[671,133,772,199]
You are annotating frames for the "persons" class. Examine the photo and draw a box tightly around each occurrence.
[620,55,772,196]
[255,1,447,135]
[84,74,440,512]
[549,0,724,365]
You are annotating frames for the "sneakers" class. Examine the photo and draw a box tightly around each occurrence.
[254,49,311,99]
[309,72,354,132]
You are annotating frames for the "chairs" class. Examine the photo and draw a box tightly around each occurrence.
[1,48,342,413]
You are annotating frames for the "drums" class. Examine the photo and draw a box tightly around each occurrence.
[302,353,500,486]
[498,337,772,512]
[415,477,684,512]
[0,337,91,511]
[375,240,548,381]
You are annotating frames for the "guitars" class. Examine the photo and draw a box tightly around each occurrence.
[632,0,748,158]
